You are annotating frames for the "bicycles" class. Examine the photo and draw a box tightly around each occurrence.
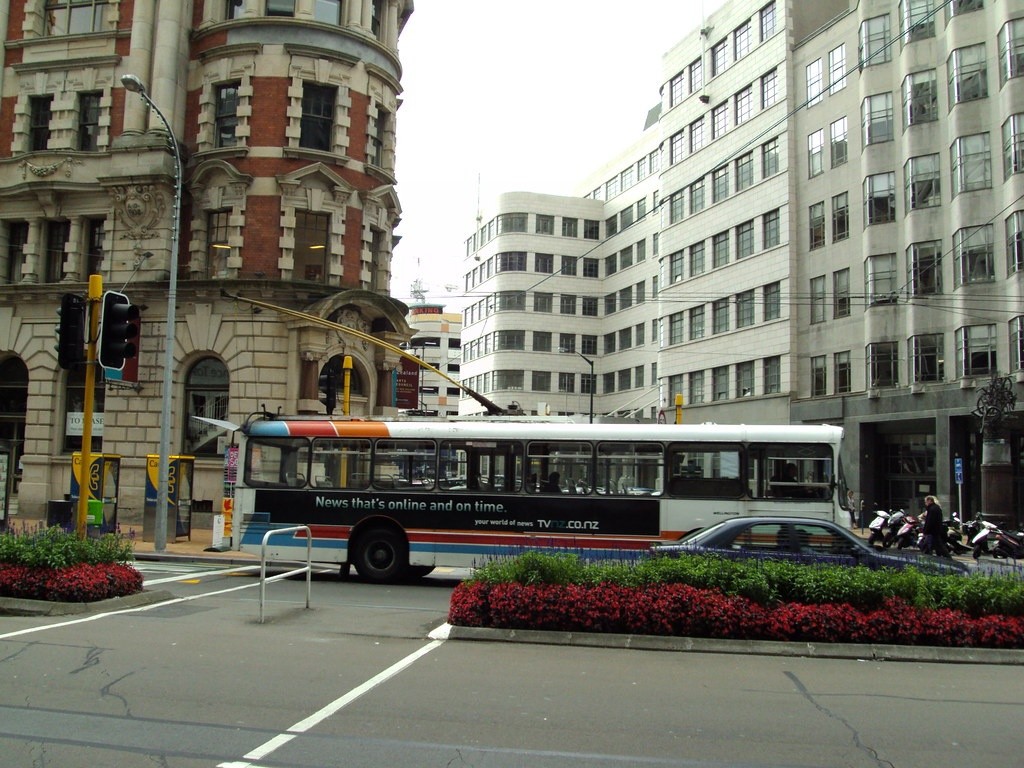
[971,372,1017,434]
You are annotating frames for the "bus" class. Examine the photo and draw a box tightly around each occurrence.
[227,408,846,586]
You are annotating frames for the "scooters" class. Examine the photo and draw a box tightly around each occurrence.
[865,505,1024,564]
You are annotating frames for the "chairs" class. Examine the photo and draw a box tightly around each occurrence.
[283,472,794,498]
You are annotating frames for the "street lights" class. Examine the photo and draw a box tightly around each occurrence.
[121,72,186,555]
[556,346,595,426]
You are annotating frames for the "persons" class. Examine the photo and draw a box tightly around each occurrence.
[781,462,816,497]
[923,497,942,558]
[928,494,952,559]
[846,490,858,529]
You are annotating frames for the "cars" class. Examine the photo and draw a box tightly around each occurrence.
[646,515,969,585]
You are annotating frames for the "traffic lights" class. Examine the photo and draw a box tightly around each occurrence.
[97,291,141,368]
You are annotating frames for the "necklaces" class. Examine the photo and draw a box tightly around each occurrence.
[543,472,561,492]
